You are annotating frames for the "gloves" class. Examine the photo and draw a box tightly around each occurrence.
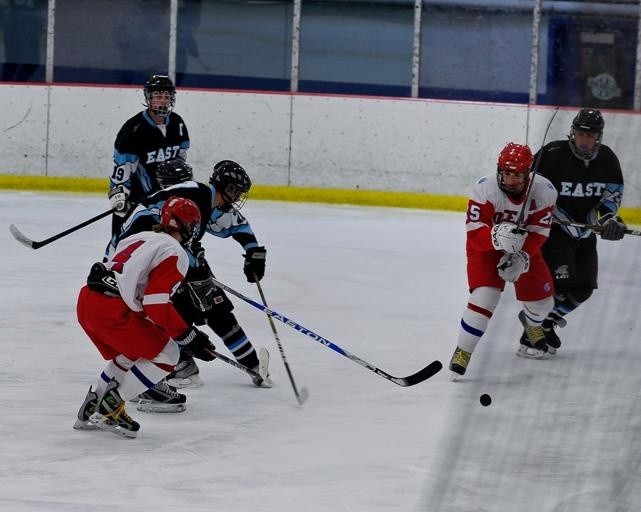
[497,250,529,282]
[491,223,528,254]
[172,326,216,363]
[239,246,267,284]
[107,187,131,218]
[600,215,628,241]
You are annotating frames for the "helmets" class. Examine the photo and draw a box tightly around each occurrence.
[569,108,605,158]
[154,159,192,189]
[210,159,252,214]
[497,142,533,194]
[160,196,201,244]
[144,74,177,118]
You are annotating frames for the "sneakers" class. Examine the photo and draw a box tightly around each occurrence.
[130,383,176,402]
[517,310,546,349]
[449,345,472,375]
[543,328,561,349]
[520,325,547,352]
[76,385,98,421]
[166,357,199,380]
[249,365,273,387]
[97,378,140,433]
[139,379,187,405]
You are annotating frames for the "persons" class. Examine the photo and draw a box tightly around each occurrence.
[102,160,266,413]
[518,109,627,358]
[73,198,215,439]
[450,143,558,375]
[109,75,190,238]
[156,160,272,389]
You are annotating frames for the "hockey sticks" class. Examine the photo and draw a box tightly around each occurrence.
[253,273,310,406]
[8,207,119,250]
[502,109,563,266]
[208,273,443,388]
